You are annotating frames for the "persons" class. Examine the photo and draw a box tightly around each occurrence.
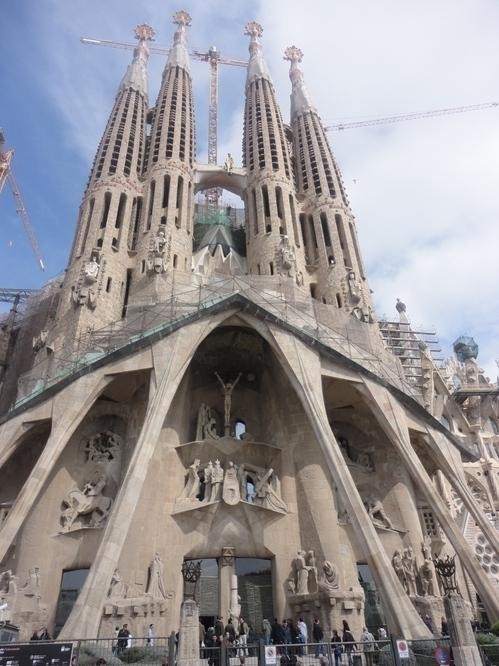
[146,552,166,599]
[84,256,101,277]
[145,623,155,648]
[78,428,122,465]
[419,613,490,638]
[179,456,288,510]
[38,628,52,641]
[224,151,235,173]
[193,402,219,442]
[214,371,243,427]
[280,550,343,593]
[30,630,39,641]
[168,615,389,666]
[151,230,169,257]
[363,492,394,529]
[110,568,132,598]
[390,545,440,599]
[77,466,106,514]
[111,623,132,658]
